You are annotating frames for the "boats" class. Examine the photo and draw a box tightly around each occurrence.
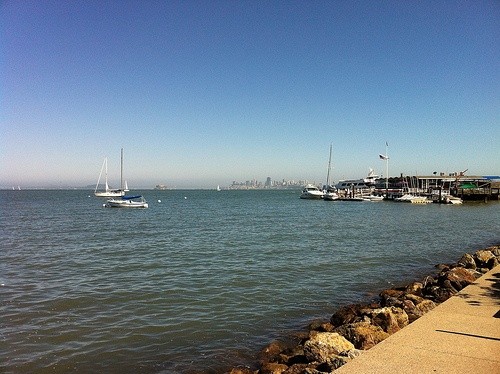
[300,184,324,200]
[319,143,463,204]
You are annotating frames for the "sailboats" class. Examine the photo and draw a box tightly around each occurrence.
[124,180,130,192]
[93,153,125,197]
[106,148,149,209]
[216,185,221,192]
